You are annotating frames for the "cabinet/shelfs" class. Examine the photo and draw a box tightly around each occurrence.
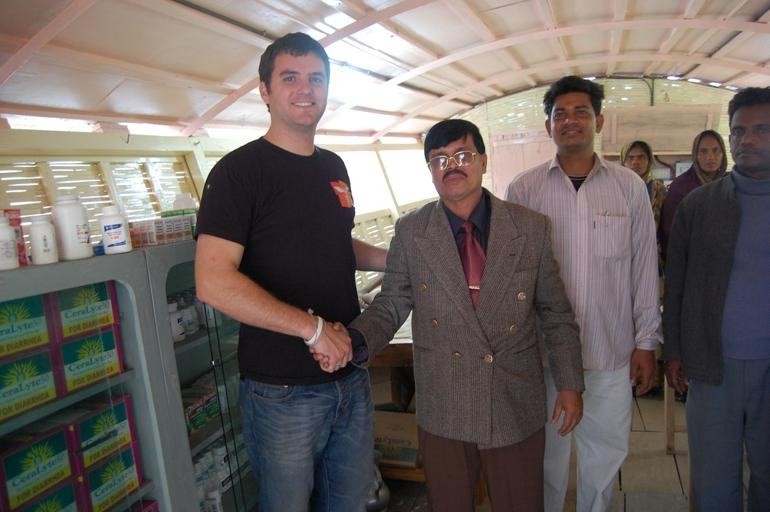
[0,251,163,512]
[146,240,258,510]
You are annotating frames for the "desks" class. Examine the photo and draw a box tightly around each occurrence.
[663,373,687,453]
[369,310,426,483]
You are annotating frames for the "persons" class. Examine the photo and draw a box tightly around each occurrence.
[309,119,586,512]
[505,76,663,512]
[658,130,732,259]
[194,32,391,512]
[618,139,667,307]
[664,87,770,512]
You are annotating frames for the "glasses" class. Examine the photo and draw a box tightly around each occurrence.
[426,150,483,171]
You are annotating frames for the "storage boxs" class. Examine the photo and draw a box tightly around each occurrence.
[374,401,422,468]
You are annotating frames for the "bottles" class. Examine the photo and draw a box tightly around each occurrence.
[192,430,252,512]
[157,192,201,240]
[0,197,133,273]
[165,285,223,344]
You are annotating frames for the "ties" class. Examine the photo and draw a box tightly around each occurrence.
[458,222,489,307]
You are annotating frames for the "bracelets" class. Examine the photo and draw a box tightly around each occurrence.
[304,316,324,347]
